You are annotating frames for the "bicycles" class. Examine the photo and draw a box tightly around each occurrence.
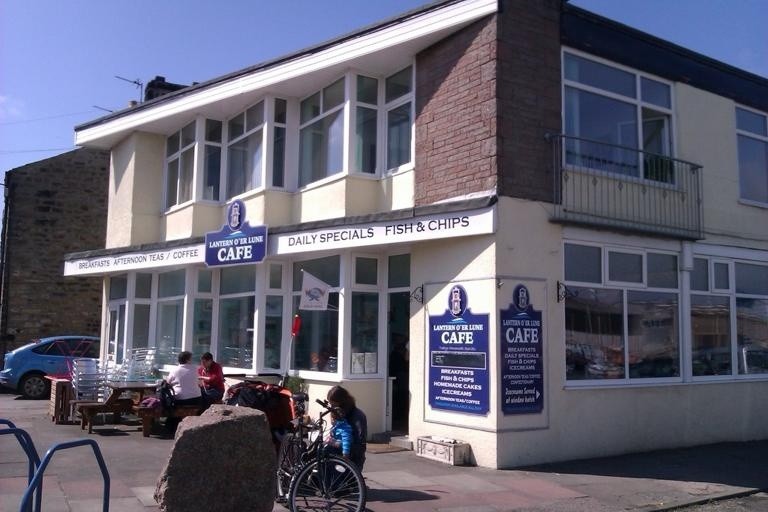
[277,392,366,512]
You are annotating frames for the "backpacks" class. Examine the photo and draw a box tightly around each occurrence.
[156,380,176,414]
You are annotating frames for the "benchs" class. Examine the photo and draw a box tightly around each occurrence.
[43,374,213,434]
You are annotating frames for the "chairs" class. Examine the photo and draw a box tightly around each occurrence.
[73,346,156,422]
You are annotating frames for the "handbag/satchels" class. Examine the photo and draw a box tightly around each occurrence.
[202,387,222,402]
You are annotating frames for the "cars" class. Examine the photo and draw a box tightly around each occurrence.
[0,335,124,399]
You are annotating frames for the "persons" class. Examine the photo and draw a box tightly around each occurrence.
[309,385,368,497]
[195,352,224,403]
[322,406,354,493]
[157,350,202,440]
